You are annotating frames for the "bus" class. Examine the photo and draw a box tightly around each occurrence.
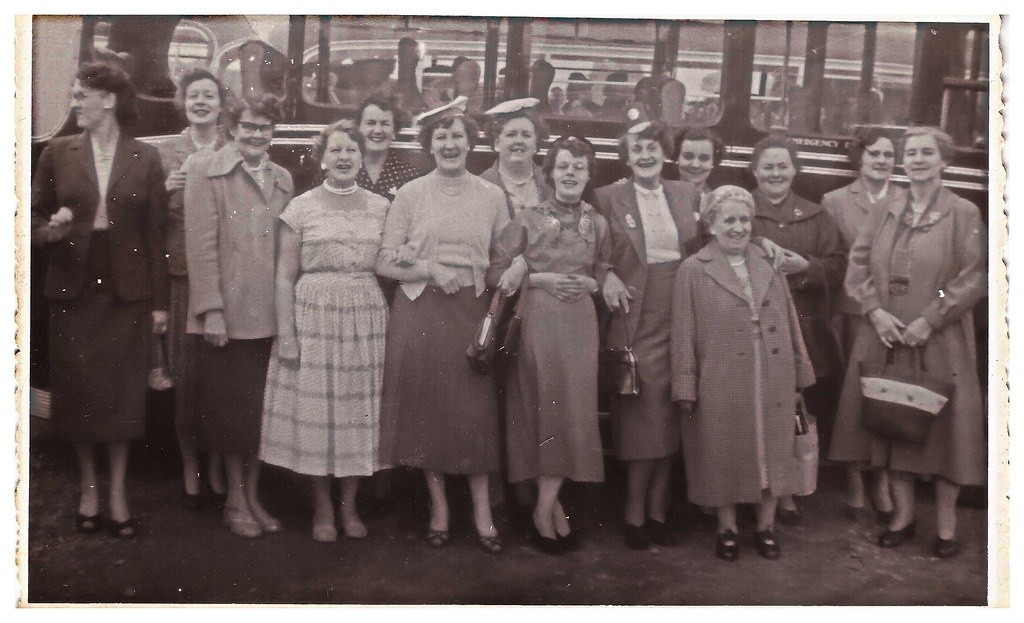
[33,16,989,243]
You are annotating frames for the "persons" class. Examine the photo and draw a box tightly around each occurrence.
[592,121,702,548]
[827,126,989,559]
[256,120,417,542]
[428,59,887,147]
[374,96,528,554]
[32,61,171,539]
[183,91,295,538]
[671,127,725,212]
[159,67,238,510]
[310,97,427,511]
[749,135,844,522]
[479,98,535,515]
[670,186,816,560]
[486,137,613,552]
[819,125,903,520]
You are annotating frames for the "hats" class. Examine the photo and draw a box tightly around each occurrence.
[483,98,540,121]
[412,97,468,125]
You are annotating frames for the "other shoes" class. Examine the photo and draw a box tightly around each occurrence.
[222,506,281,538]
[844,484,864,521]
[313,512,338,544]
[624,511,674,553]
[181,480,226,510]
[777,502,802,526]
[871,493,896,520]
[933,521,959,558]
[876,492,917,546]
[530,511,576,555]
[338,511,366,539]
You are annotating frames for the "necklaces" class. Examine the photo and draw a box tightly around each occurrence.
[323,178,358,195]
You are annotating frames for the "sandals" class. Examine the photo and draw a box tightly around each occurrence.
[108,509,137,538]
[755,529,778,559]
[425,511,451,551]
[717,529,737,564]
[477,528,503,554]
[75,502,100,533]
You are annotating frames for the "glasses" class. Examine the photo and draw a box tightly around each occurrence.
[236,121,273,133]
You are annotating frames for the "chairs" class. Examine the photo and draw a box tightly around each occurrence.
[236,39,685,129]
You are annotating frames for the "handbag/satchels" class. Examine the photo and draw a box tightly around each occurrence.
[786,398,819,496]
[465,287,506,378]
[494,274,527,359]
[599,306,640,399]
[800,254,847,373]
[858,334,949,444]
